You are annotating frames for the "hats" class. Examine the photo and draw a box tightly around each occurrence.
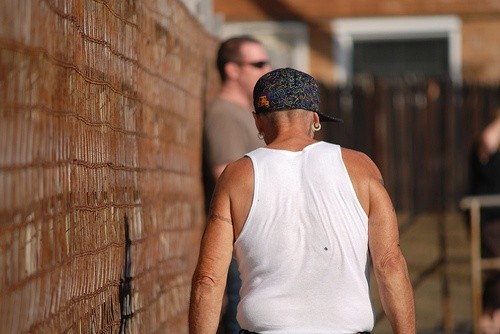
[252,67,343,124]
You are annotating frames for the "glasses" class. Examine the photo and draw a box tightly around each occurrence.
[240,61,269,69]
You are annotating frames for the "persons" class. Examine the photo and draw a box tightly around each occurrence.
[202,35,274,182]
[187,67,416,334]
[453,114,500,334]
